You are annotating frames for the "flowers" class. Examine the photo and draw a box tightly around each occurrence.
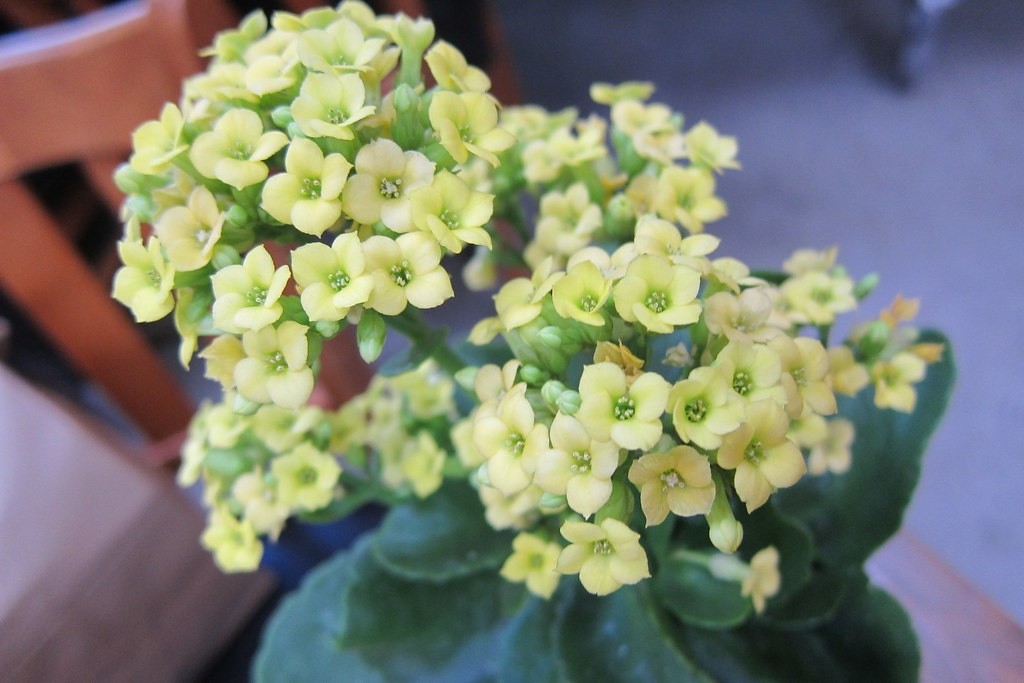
[114,0,957,681]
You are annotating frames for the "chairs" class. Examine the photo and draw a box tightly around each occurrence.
[114,0,964,683]
[0,1,372,552]
